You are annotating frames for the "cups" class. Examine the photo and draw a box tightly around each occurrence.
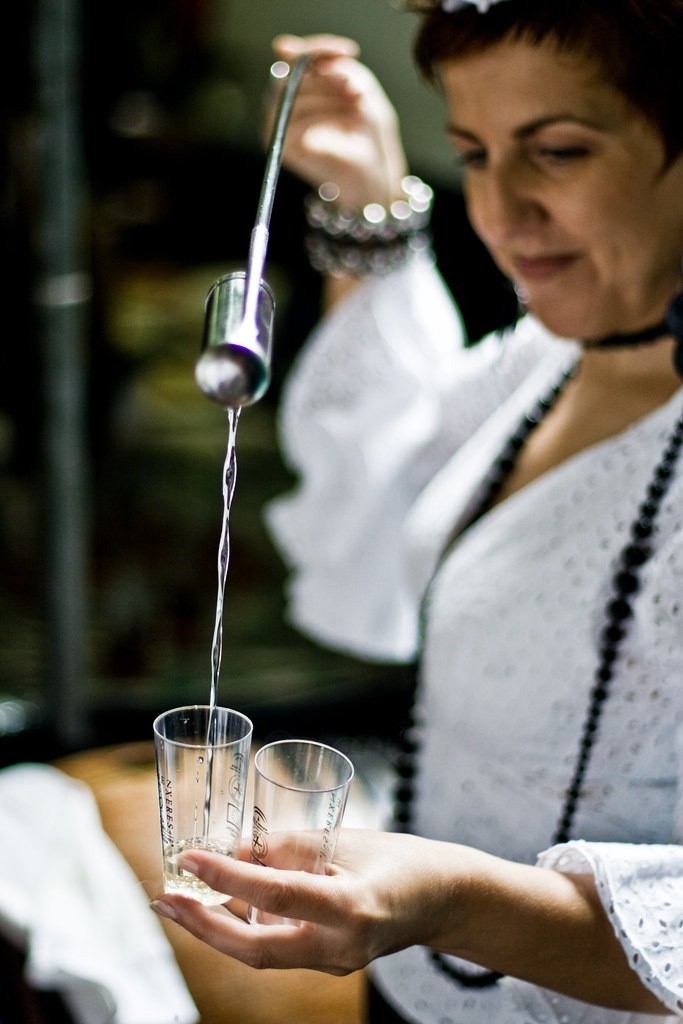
[246,738,355,924]
[153,705,254,906]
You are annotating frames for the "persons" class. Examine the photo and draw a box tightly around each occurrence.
[147,0,683,1024]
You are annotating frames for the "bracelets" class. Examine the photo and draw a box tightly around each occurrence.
[300,174,433,278]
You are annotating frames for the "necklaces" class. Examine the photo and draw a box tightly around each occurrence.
[385,289,683,990]
